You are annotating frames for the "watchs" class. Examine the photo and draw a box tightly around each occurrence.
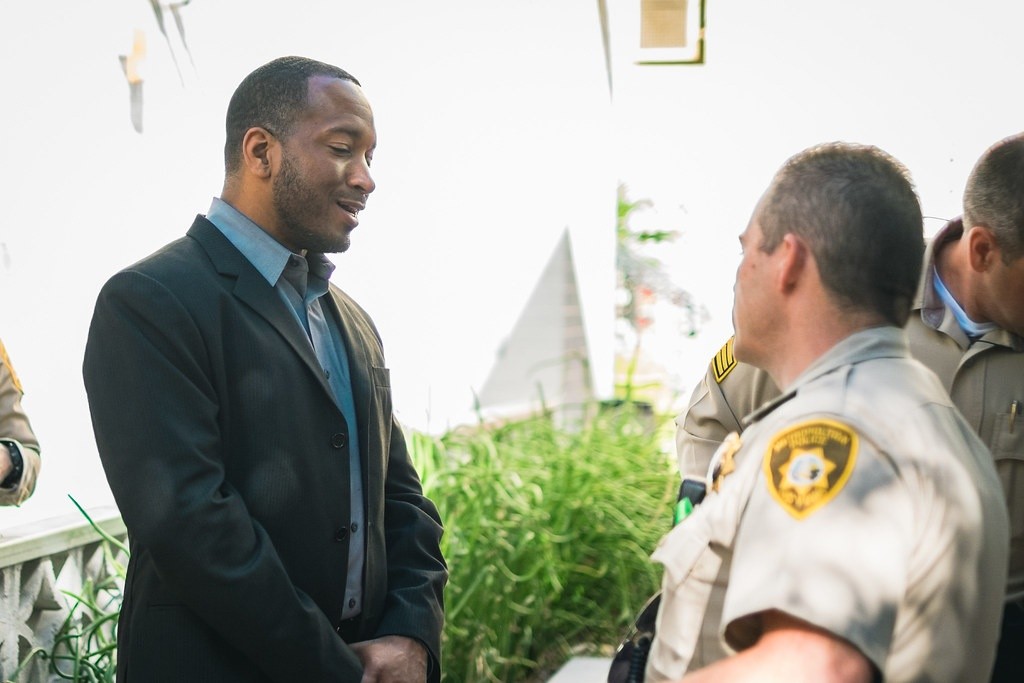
[1,441,23,487]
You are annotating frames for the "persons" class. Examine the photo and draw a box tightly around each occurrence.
[675,133,1024,683]
[643,141,1011,683]
[0,338,41,507]
[82,57,448,683]
